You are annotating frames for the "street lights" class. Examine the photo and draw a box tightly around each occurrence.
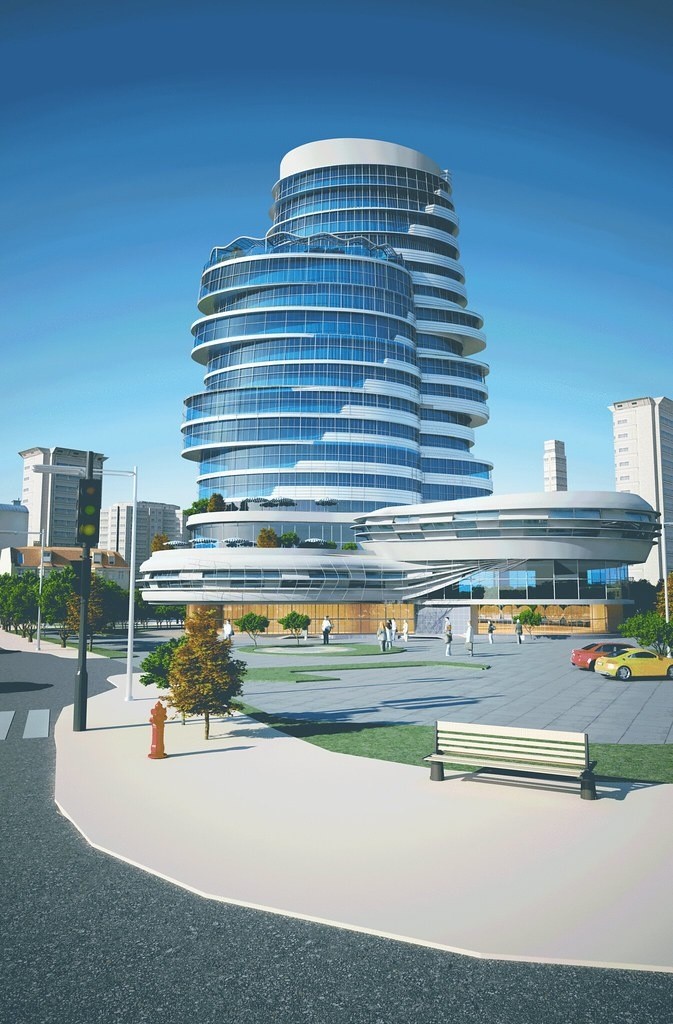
[31,465,137,700]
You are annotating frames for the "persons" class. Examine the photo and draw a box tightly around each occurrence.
[488,620,495,643]
[322,616,331,644]
[515,619,523,644]
[465,620,474,657]
[444,617,452,657]
[377,618,408,651]
[224,618,233,644]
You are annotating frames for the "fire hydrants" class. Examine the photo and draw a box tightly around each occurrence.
[148,701,169,759]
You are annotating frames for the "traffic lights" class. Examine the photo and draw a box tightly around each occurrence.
[75,478,101,546]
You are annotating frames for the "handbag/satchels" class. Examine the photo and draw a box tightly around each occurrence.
[520,635,525,640]
[465,642,472,650]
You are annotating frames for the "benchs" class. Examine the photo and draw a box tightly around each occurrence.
[421,719,597,800]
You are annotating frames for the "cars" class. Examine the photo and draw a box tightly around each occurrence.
[570,642,635,672]
[594,648,673,681]
